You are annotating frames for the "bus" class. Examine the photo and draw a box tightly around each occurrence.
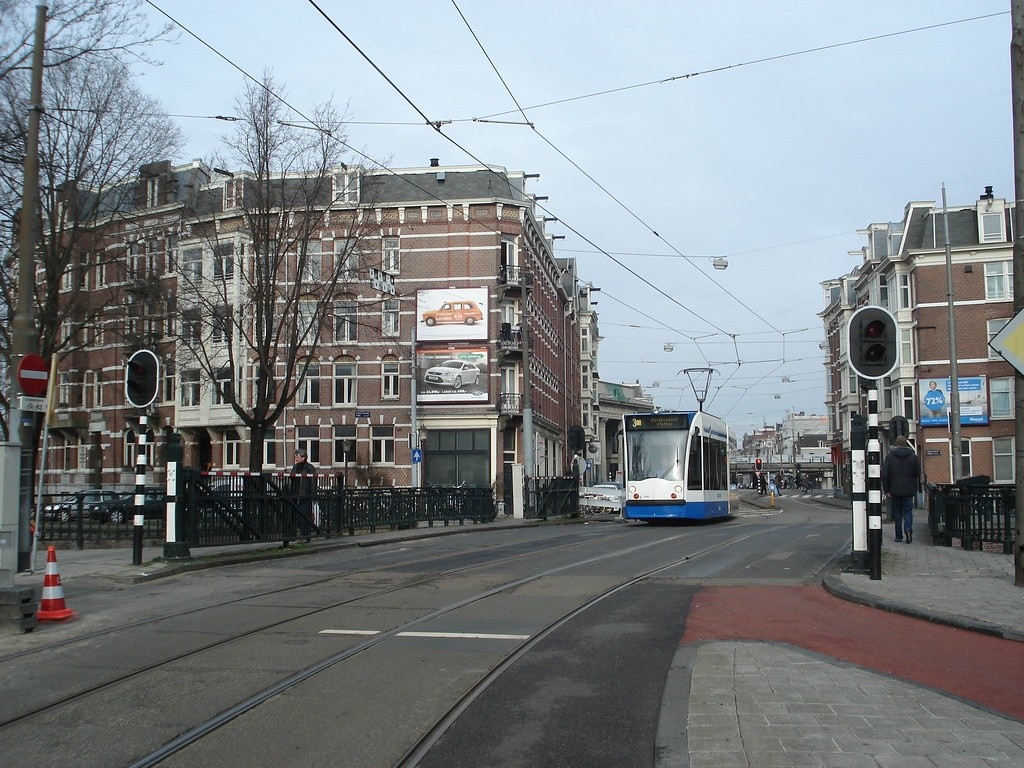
[621,410,729,523]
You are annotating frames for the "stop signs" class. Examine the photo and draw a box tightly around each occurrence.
[18,355,48,396]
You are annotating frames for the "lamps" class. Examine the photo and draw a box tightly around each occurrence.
[988,198,993,205]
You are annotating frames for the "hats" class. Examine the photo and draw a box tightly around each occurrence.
[895,435,907,446]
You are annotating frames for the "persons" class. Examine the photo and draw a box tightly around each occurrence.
[774,472,782,496]
[288,449,316,544]
[760,473,768,495]
[926,381,944,418]
[880,435,920,544]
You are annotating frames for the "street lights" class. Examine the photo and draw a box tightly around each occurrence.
[417,424,429,487]
[342,438,352,487]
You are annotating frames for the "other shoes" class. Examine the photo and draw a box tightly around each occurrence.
[905,531,912,543]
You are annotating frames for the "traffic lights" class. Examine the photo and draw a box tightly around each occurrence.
[848,305,899,380]
[125,350,160,408]
[795,463,798,470]
[798,463,801,470]
[780,469,784,476]
[756,459,763,471]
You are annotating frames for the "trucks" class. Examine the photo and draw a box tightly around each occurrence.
[201,479,268,518]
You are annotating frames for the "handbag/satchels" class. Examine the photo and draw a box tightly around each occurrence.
[313,501,319,527]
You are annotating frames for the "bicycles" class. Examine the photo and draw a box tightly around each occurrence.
[579,496,614,519]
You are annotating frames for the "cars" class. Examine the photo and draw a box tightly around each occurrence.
[45,490,120,523]
[424,360,480,389]
[422,301,484,327]
[590,482,625,513]
[90,487,167,524]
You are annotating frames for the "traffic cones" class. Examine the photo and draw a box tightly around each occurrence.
[37,546,77,621]
